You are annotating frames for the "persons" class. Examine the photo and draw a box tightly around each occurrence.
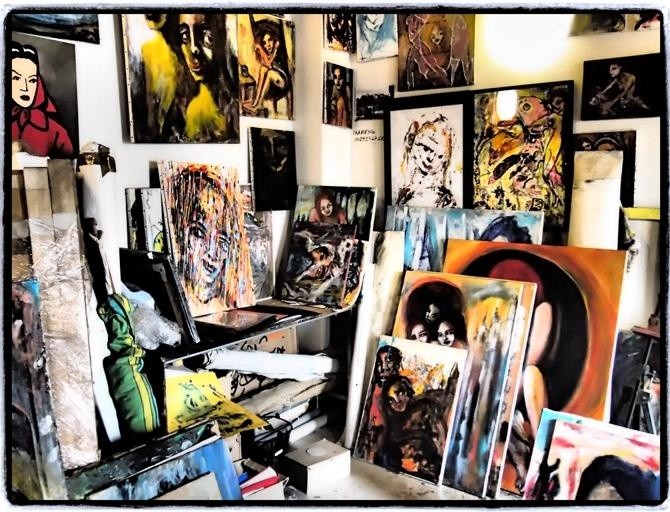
[3,8,664,505]
